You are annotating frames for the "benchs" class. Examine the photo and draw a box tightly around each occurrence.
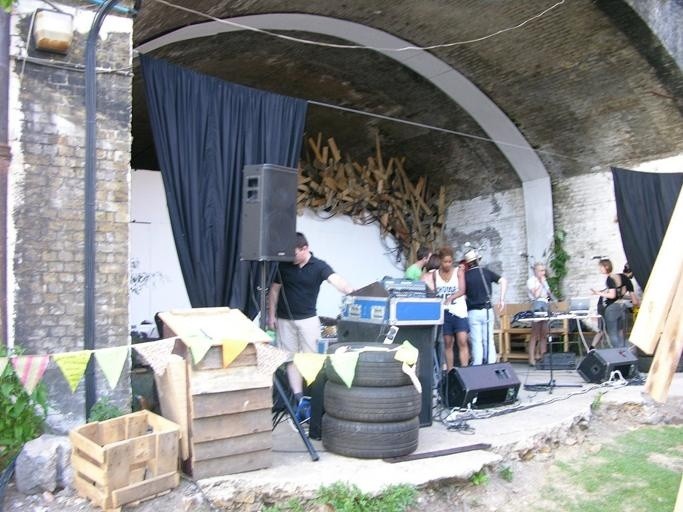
[492,302,568,362]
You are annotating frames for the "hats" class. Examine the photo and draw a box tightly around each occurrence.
[462,247,483,266]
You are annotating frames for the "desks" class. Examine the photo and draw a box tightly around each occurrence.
[518,315,602,353]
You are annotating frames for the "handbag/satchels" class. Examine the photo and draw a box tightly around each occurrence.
[478,266,503,331]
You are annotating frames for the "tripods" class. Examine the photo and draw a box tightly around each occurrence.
[260,264,320,462]
[524,257,583,394]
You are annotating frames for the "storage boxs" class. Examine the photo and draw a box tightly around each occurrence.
[70,408,181,512]
[343,281,445,328]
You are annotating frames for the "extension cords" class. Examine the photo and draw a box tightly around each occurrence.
[383,325,399,345]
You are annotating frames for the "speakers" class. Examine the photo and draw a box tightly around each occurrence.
[437,362,522,408]
[576,346,639,384]
[239,163,298,263]
[336,320,433,429]
[535,350,577,370]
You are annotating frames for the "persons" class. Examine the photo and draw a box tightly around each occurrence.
[525,262,561,367]
[583,261,640,352]
[406,245,506,371]
[267,231,356,404]
[589,259,627,348]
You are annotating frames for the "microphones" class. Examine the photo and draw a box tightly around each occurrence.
[519,251,534,258]
[465,242,478,250]
